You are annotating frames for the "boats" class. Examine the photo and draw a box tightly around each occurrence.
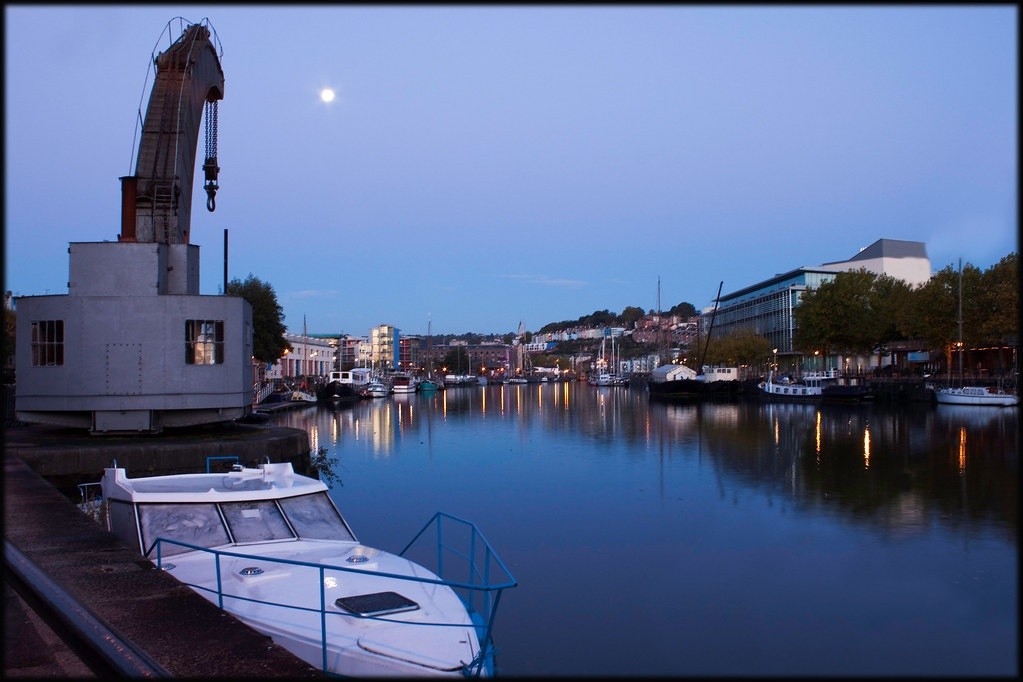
[320,330,367,399]
[77,452,518,680]
[365,346,390,398]
[757,366,868,404]
[647,364,746,396]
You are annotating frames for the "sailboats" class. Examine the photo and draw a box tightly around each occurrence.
[933,257,1019,405]
[444,346,476,386]
[393,337,418,393]
[419,317,441,391]
[290,313,317,403]
[589,335,630,387]
